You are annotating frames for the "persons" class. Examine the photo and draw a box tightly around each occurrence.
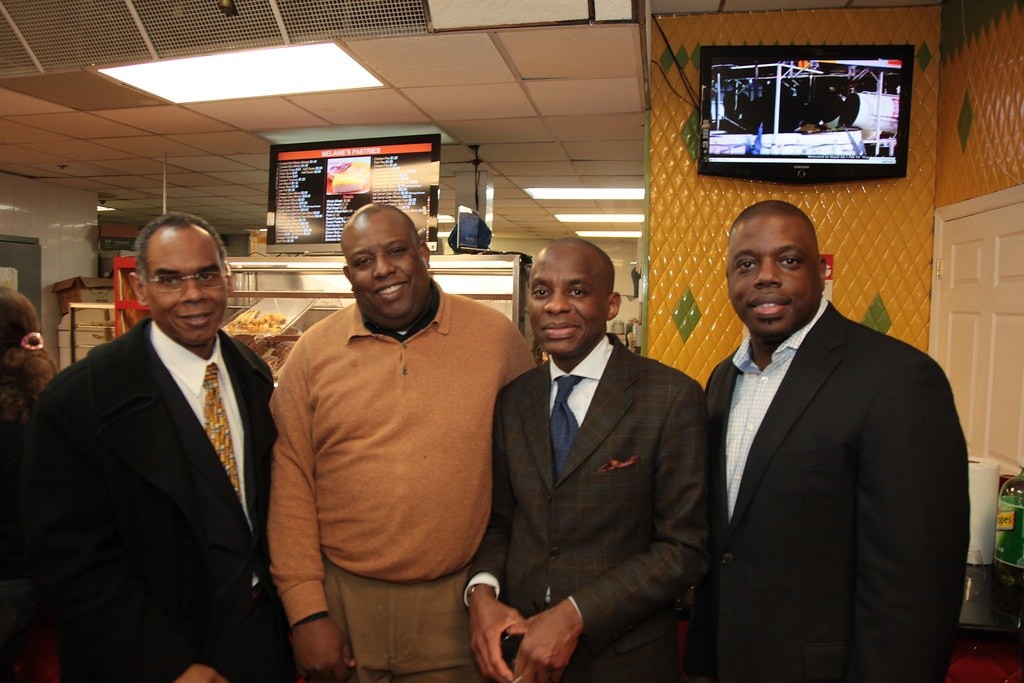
[265,205,538,683]
[0,285,57,683]
[705,199,972,683]
[460,237,706,683]
[25,210,299,683]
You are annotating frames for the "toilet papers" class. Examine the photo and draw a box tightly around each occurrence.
[967,455,999,565]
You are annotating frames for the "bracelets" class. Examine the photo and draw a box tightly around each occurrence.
[466,583,478,594]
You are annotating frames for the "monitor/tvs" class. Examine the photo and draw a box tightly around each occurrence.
[698,44,915,186]
[267,132,441,253]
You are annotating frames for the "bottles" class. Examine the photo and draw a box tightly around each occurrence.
[989,457,1024,630]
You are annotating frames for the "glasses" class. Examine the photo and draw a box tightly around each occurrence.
[140,272,229,292]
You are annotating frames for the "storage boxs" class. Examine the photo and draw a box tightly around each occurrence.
[52,276,115,315]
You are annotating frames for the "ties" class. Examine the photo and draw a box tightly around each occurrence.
[202,363,242,502]
[549,375,584,485]
